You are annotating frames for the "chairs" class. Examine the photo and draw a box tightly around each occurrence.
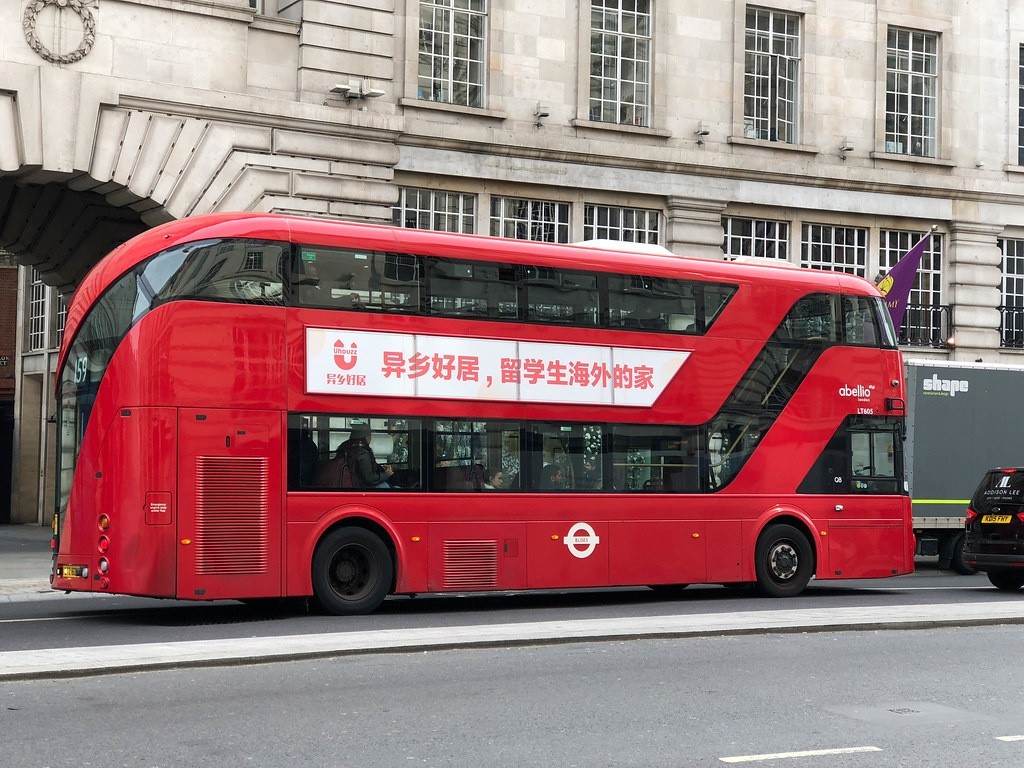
[305,451,667,494]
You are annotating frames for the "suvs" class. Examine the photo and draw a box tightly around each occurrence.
[962,467,1024,593]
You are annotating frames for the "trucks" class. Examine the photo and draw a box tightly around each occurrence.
[854,359,1024,575]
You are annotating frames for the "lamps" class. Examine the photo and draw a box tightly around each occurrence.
[838,135,856,150]
[976,161,985,166]
[329,85,360,99]
[695,120,710,136]
[360,78,387,98]
[536,100,552,118]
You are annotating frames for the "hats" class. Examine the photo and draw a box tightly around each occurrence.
[350,422,371,439]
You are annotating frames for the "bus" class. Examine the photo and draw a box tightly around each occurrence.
[49,209,914,617]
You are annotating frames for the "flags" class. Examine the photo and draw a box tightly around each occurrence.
[876,237,931,339]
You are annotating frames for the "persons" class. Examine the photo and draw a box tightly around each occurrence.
[299,266,357,309]
[287,417,599,494]
[625,303,669,331]
